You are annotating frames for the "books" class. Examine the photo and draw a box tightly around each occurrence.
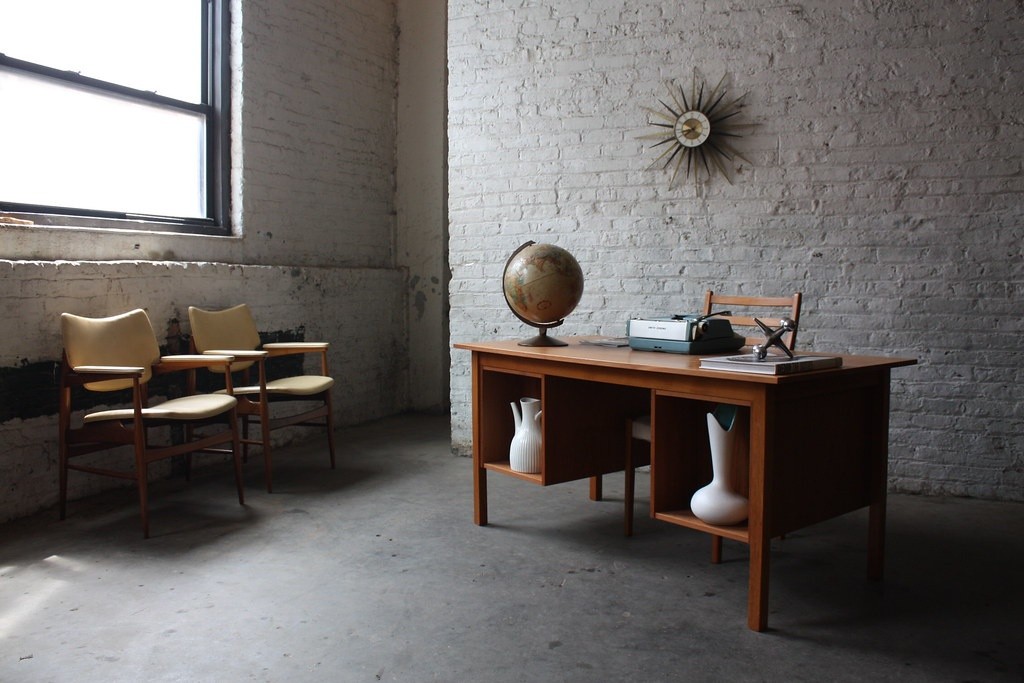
[699,350,843,376]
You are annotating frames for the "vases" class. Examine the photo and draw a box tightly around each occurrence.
[690,404,750,526]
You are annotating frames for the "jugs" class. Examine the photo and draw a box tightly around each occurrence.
[510,397,543,473]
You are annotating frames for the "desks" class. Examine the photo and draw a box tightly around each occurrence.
[451,336,920,630]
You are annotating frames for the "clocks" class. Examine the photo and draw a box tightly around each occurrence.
[634,67,760,197]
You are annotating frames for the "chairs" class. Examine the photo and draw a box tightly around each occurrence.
[57,307,245,539]
[623,289,802,563]
[183,303,336,492]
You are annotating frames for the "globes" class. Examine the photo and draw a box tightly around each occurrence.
[502,240,584,347]
